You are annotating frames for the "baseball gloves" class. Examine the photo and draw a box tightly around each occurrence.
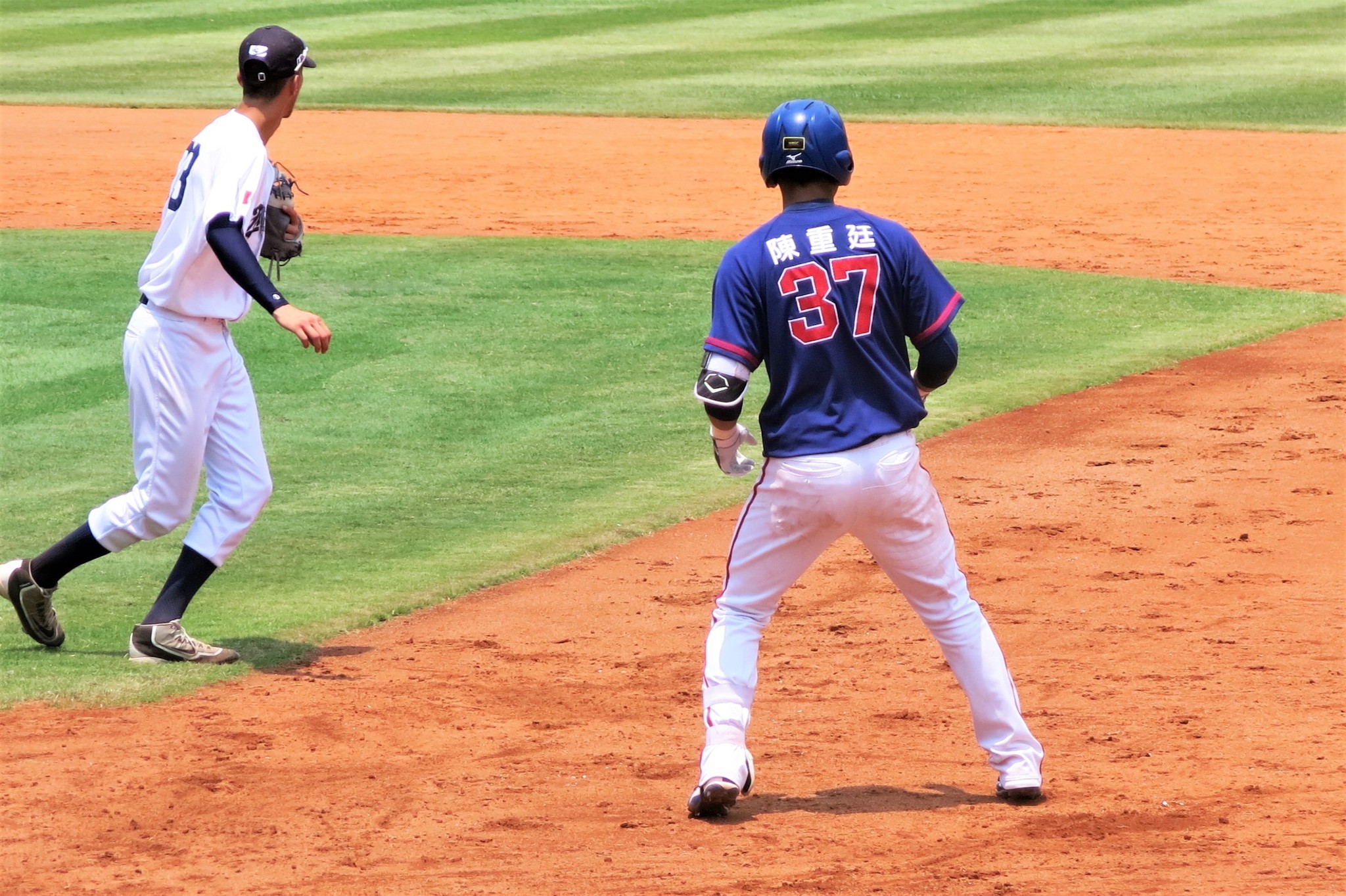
[257,158,311,284]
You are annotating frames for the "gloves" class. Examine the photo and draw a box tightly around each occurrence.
[910,367,936,402]
[710,423,757,477]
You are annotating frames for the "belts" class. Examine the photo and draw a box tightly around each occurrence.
[140,293,149,305]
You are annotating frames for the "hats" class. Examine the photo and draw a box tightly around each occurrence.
[239,25,316,86]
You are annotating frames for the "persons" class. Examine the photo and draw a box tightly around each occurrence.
[0,26,334,666]
[689,98,1047,821]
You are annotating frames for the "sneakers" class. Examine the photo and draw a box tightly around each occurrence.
[128,619,240,665]
[996,777,1041,798]
[688,776,740,819]
[0,559,66,647]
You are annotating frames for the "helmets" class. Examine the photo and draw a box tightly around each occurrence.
[759,99,854,188]
